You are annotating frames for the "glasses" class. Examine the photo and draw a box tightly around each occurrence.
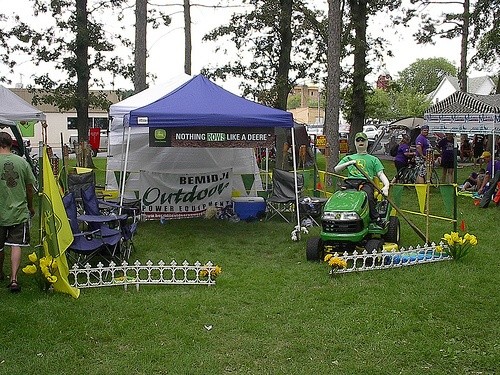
[356,138,365,142]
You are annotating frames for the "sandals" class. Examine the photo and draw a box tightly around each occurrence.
[8,280,18,289]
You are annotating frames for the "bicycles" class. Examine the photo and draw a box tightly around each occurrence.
[398,154,440,190]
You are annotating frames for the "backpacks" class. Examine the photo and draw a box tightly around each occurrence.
[390,144,399,157]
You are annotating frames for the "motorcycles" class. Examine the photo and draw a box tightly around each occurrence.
[306,178,400,266]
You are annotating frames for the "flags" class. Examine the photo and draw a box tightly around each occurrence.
[38,145,86,298]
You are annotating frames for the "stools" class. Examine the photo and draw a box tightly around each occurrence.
[300,196,328,227]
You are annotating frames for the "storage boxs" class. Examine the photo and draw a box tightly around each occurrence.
[230,197,265,220]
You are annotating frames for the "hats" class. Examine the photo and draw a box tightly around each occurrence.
[355,132,368,140]
[480,151,491,158]
[421,125,429,130]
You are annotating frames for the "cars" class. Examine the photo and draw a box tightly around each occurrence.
[362,125,389,146]
[10,138,32,158]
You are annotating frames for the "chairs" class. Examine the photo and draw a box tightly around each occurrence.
[62,167,141,281]
[265,168,304,223]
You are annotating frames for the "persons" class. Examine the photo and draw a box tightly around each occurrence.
[477,152,500,208]
[392,133,417,186]
[335,132,390,222]
[436,133,457,184]
[415,124,430,184]
[460,133,500,193]
[0,132,38,290]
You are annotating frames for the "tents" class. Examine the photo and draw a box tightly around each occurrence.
[102,69,263,211]
[117,71,305,240]
[0,84,48,153]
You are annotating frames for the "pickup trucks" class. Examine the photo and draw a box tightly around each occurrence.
[70,129,108,157]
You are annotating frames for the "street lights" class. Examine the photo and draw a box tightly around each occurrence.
[318,87,323,124]
